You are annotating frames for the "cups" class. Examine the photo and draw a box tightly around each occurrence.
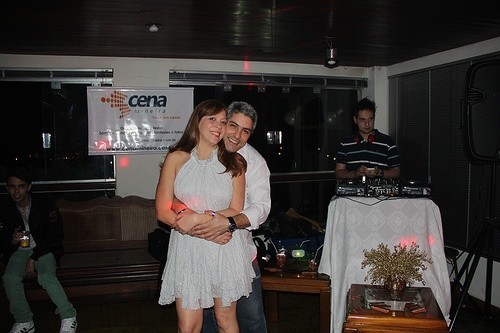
[309,260,317,279]
[19,231,30,248]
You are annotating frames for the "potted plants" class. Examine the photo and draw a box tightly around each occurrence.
[361,241,433,301]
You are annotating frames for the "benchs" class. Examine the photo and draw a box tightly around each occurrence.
[23,196,159,311]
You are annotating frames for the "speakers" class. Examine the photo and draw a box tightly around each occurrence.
[463,59,500,164]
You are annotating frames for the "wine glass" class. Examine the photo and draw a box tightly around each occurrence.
[277,254,286,276]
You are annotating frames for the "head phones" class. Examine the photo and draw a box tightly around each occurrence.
[353,128,376,144]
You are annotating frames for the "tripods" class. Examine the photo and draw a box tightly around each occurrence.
[449,167,500,333]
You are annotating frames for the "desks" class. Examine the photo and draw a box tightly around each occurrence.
[262,271,331,333]
[342,284,449,333]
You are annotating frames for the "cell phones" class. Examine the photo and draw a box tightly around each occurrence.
[366,168,376,170]
